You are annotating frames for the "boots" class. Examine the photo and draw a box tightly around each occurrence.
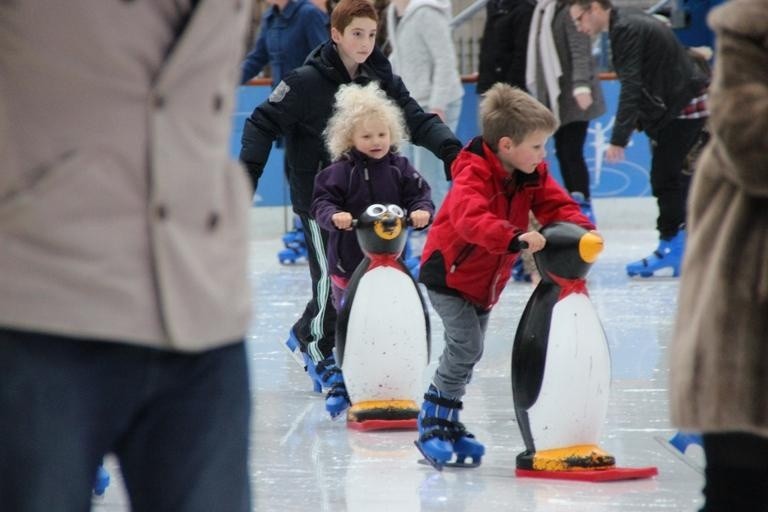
[624,226,686,277]
[670,427,705,455]
[276,217,309,264]
[94,464,112,496]
[447,397,485,458]
[416,378,455,462]
[571,189,595,225]
[289,325,350,413]
[511,256,528,281]
